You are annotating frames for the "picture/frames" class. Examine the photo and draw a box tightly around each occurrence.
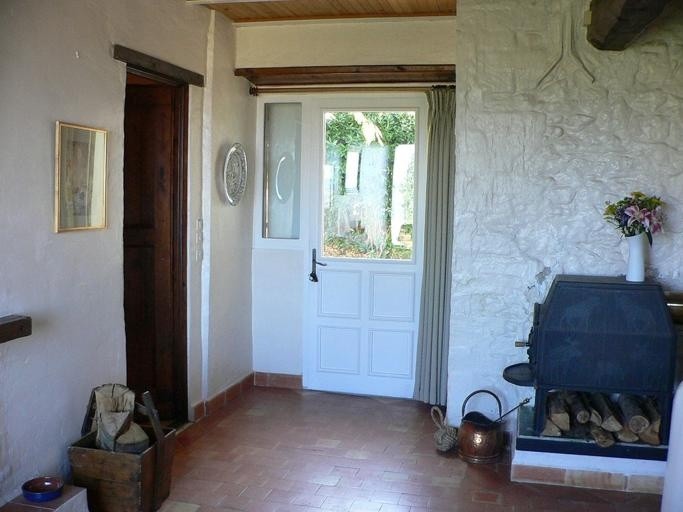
[53,119,109,235]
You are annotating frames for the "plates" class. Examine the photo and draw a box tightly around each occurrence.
[221,141,248,206]
[274,151,294,203]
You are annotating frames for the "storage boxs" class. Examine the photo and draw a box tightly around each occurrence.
[0,385,178,512]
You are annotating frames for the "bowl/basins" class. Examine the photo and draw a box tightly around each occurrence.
[21,476,64,503]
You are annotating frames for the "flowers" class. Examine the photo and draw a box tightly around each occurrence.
[603,188,666,247]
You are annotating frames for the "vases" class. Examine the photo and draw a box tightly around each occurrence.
[624,231,649,283]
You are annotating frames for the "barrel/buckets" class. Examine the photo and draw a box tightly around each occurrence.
[456,390,506,465]
